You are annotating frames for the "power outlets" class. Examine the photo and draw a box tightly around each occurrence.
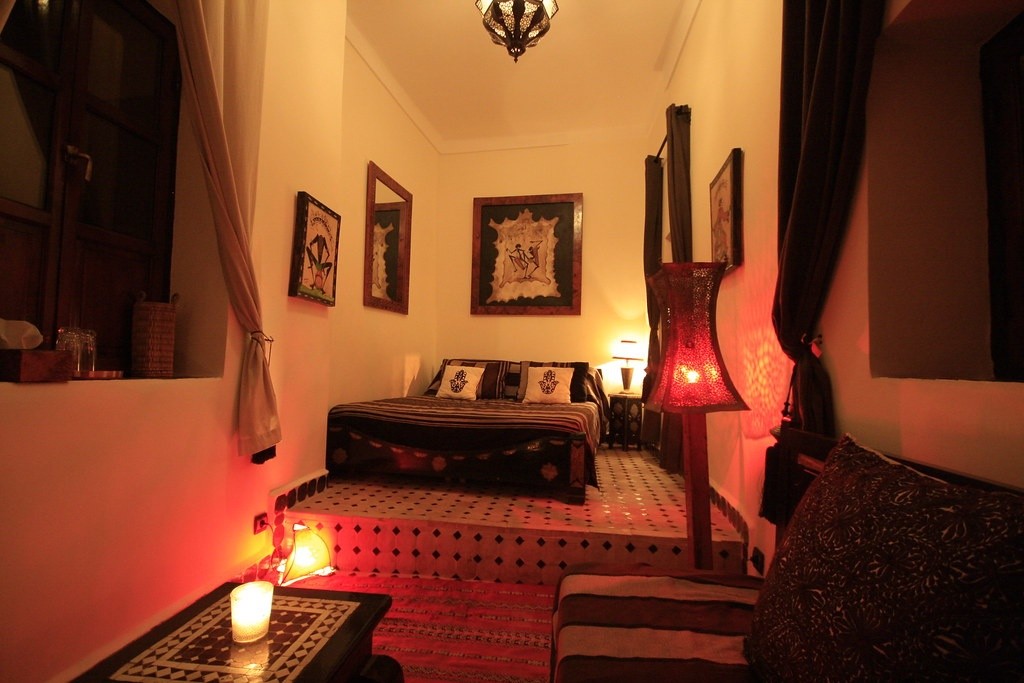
[253,512,269,536]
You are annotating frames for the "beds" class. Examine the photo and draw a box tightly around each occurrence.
[324,357,607,504]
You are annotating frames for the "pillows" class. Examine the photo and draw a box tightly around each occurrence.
[429,359,510,398]
[523,367,573,404]
[515,359,589,403]
[435,366,486,400]
[746,433,1024,683]
[428,364,597,401]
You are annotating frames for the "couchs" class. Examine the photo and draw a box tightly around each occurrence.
[551,439,1024,683]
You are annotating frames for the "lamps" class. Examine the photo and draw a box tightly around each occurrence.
[475,0,559,64]
[611,340,645,393]
[230,520,339,642]
[641,261,741,570]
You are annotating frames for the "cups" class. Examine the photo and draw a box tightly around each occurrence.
[230,581,274,643]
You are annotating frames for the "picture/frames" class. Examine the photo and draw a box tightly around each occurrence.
[470,191,584,317]
[706,145,745,274]
[373,202,404,302]
[288,190,341,307]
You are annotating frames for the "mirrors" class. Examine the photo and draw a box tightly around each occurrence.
[363,160,414,315]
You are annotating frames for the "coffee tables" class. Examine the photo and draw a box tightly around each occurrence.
[65,578,405,683]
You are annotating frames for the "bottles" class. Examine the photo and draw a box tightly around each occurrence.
[56,327,96,371]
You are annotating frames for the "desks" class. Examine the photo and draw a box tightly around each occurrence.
[606,394,647,451]
